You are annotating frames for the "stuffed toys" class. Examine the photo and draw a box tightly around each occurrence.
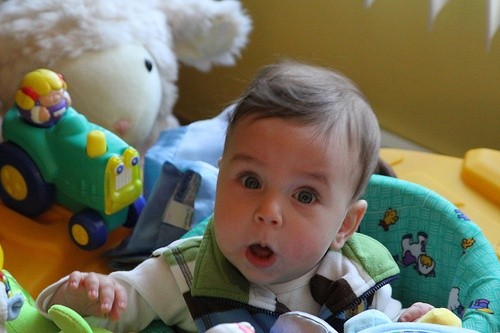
[0,0,254,182]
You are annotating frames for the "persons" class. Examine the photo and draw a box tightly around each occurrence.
[33,62,434,332]
[15,68,72,127]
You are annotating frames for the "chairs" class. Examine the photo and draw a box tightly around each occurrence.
[179,168,499,333]
